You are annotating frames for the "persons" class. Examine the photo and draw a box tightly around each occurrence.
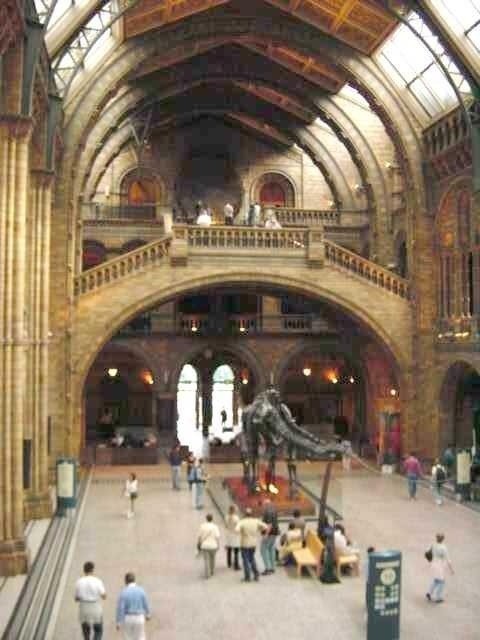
[115,572,152,640]
[342,439,352,470]
[75,562,107,639]
[112,432,124,448]
[144,432,158,447]
[214,432,266,460]
[426,532,453,603]
[431,448,473,503]
[170,441,208,509]
[198,499,361,582]
[195,199,282,229]
[126,473,138,515]
[221,409,227,425]
[100,409,112,424]
[404,452,423,502]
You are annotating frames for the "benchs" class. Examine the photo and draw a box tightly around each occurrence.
[289,530,327,576]
[326,530,363,578]
